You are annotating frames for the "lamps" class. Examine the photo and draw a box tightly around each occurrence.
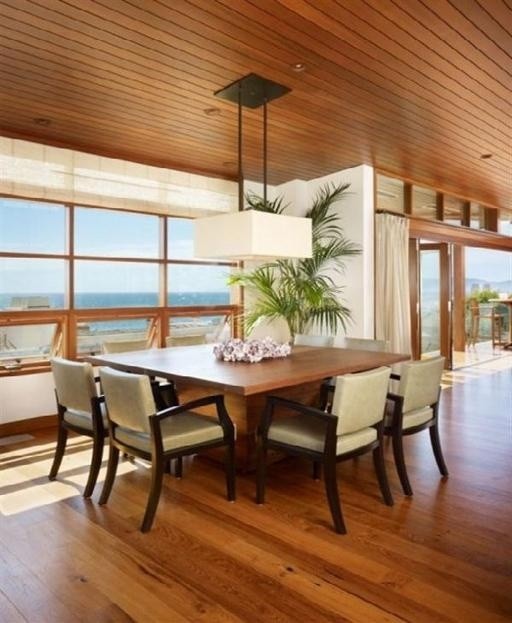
[194,72,313,259]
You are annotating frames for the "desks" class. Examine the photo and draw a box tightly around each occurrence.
[489,298,512,349]
[87,341,410,472]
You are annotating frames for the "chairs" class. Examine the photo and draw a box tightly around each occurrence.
[294,333,337,346]
[256,366,393,531]
[469,298,501,351]
[49,357,183,499]
[329,355,447,496]
[96,367,239,533]
[346,336,388,350]
